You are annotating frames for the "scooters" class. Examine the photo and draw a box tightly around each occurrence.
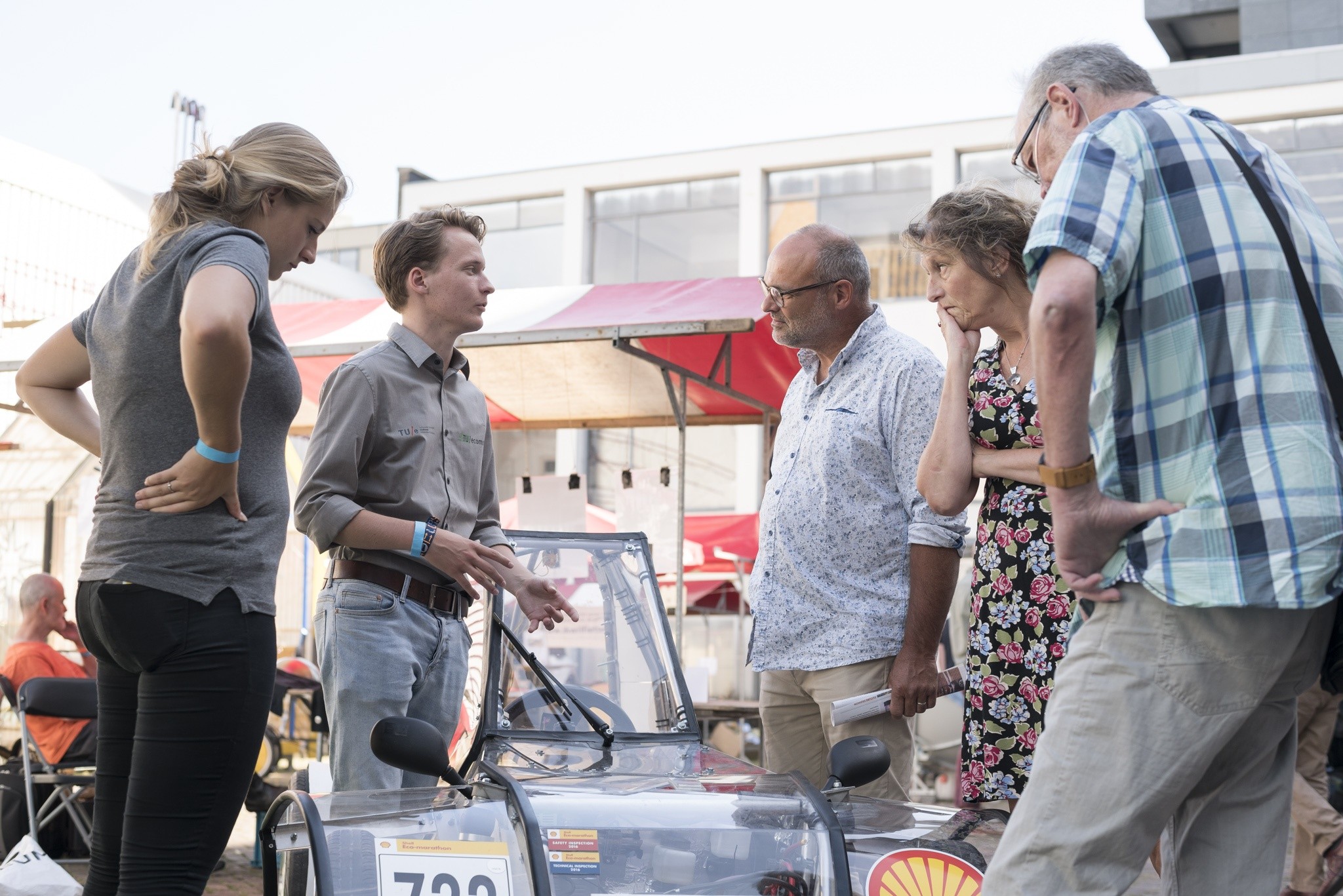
[254,528,891,895]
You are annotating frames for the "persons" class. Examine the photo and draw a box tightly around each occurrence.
[12,121,355,896]
[743,221,971,832]
[294,202,583,822]
[901,189,1160,875]
[978,42,1343,894]
[1291,670,1343,896]
[0,573,290,873]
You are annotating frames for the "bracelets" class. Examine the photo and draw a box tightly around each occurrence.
[193,438,242,464]
[1035,453,1096,489]
[419,515,440,556]
[81,649,93,659]
[410,520,426,559]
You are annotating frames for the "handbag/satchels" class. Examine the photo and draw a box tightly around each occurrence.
[1,758,63,863]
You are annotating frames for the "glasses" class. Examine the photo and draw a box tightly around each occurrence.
[757,277,844,306]
[1012,84,1076,185]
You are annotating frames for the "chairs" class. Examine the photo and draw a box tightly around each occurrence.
[0,673,109,774]
[17,676,110,862]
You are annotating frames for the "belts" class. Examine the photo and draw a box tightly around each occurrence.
[326,556,472,624]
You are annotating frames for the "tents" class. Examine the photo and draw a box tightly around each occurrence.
[265,270,800,702]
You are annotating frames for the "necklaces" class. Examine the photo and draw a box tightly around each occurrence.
[1002,337,1030,387]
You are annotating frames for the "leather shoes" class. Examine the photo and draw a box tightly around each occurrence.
[244,773,289,811]
[210,859,226,872]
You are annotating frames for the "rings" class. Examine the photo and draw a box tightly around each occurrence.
[168,481,174,493]
[917,701,927,707]
[937,321,942,327]
